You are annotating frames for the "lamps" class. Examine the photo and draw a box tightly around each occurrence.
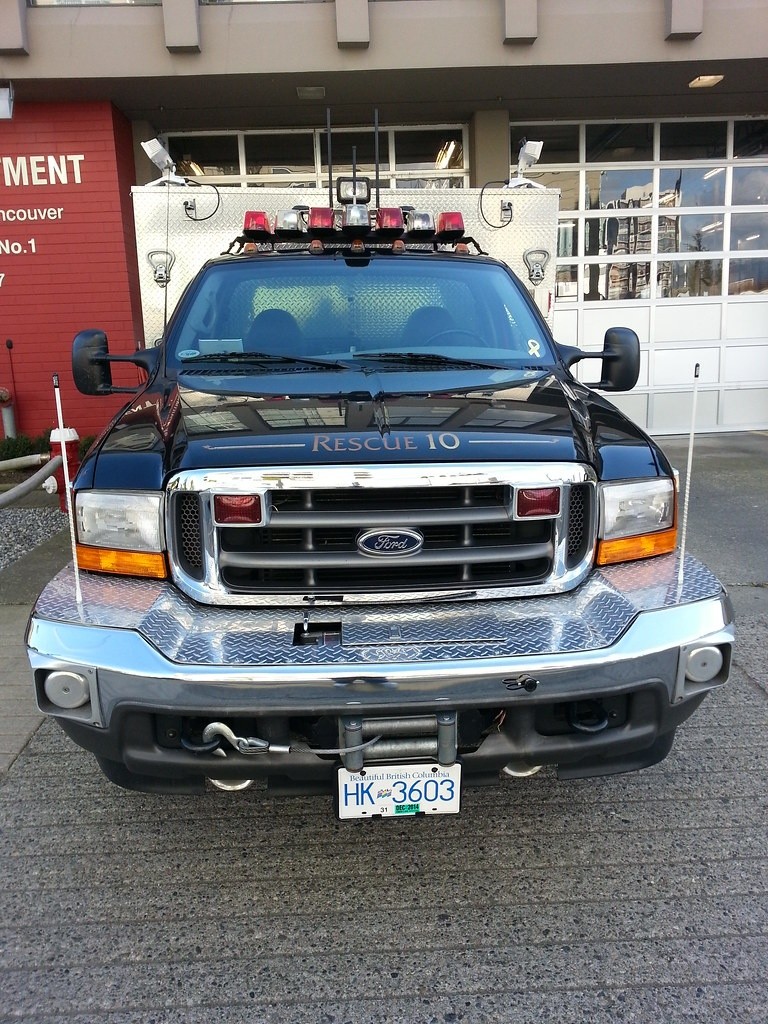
[516,141,544,178]
[0,80,15,119]
[141,138,177,177]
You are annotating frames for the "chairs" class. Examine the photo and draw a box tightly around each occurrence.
[242,309,309,359]
[397,307,462,345]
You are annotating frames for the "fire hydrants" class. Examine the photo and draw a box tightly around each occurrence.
[42,426,81,512]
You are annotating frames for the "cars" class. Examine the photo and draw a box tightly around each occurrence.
[26,200,741,800]
